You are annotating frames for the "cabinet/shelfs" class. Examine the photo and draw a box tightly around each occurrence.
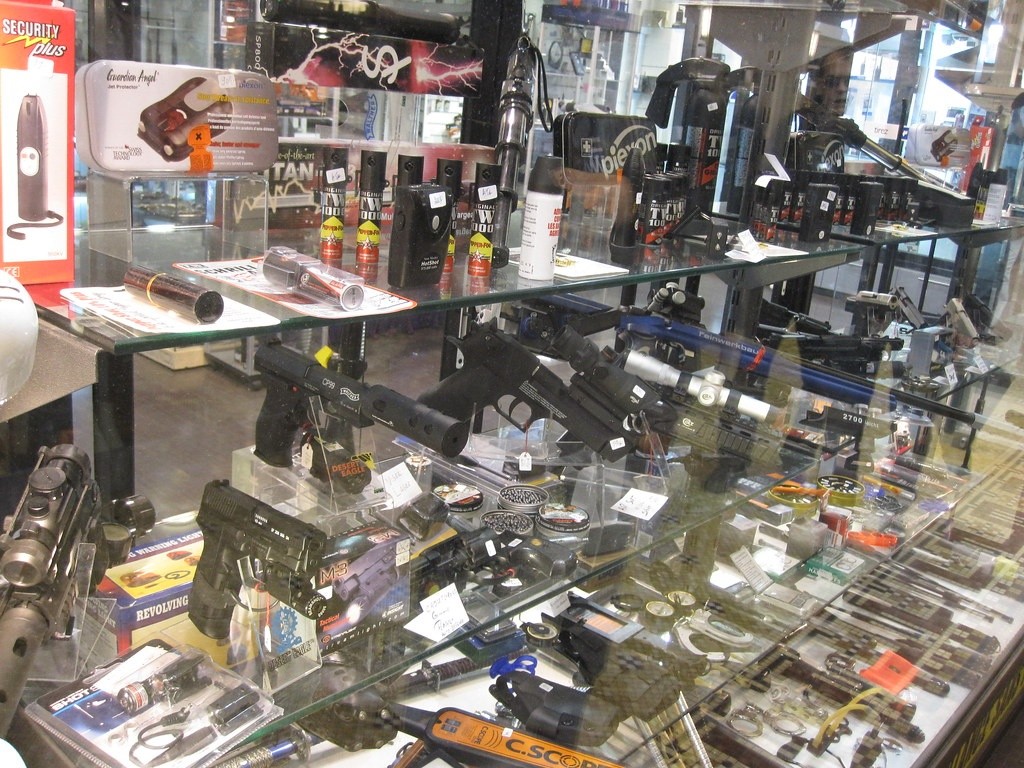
[1,0,1024,768]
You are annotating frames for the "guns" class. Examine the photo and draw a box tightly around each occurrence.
[0,0,1024,767]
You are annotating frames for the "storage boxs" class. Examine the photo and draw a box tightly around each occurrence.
[73,528,259,675]
[0,0,76,287]
[266,521,409,661]
